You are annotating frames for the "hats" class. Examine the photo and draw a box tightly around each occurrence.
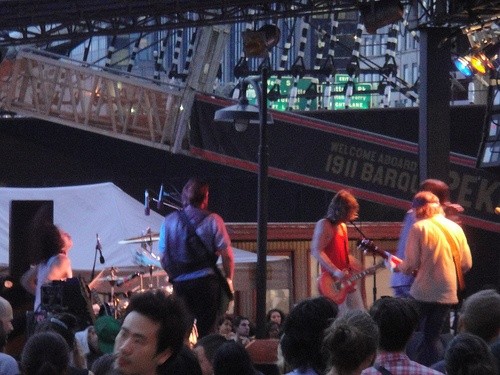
[94,315,121,353]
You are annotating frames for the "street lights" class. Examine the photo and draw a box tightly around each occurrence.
[215,61,270,338]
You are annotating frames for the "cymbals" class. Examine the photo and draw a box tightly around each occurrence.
[119,234,160,244]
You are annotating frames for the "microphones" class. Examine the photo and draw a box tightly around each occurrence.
[116,273,138,287]
[157,183,164,210]
[97,234,105,263]
[144,189,150,215]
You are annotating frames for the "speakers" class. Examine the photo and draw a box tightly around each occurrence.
[9,200,54,276]
[244,340,285,375]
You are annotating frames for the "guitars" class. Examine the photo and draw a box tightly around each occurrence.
[318,262,385,304]
[357,239,419,277]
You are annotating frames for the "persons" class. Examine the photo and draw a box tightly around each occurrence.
[319,310,380,375]
[459,288,500,355]
[159,178,235,342]
[445,331,500,375]
[363,295,441,375]
[236,317,255,349]
[390,179,451,298]
[111,288,190,375]
[194,316,283,375]
[263,324,286,341]
[34,233,122,375]
[266,309,285,323]
[396,192,472,368]
[311,190,367,314]
[1,297,18,371]
[283,297,339,375]
